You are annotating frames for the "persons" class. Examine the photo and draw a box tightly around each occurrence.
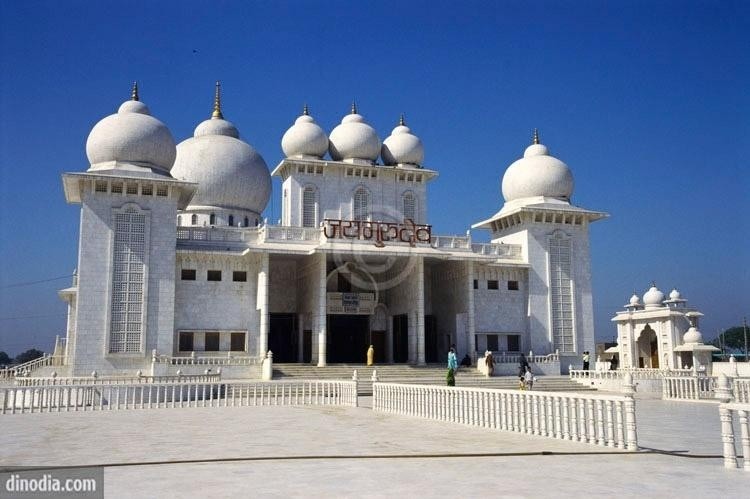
[525,366,536,391]
[519,376,525,391]
[582,351,586,358]
[366,344,374,366]
[518,352,531,382]
[484,351,496,379]
[580,350,590,371]
[604,354,619,370]
[445,343,459,387]
